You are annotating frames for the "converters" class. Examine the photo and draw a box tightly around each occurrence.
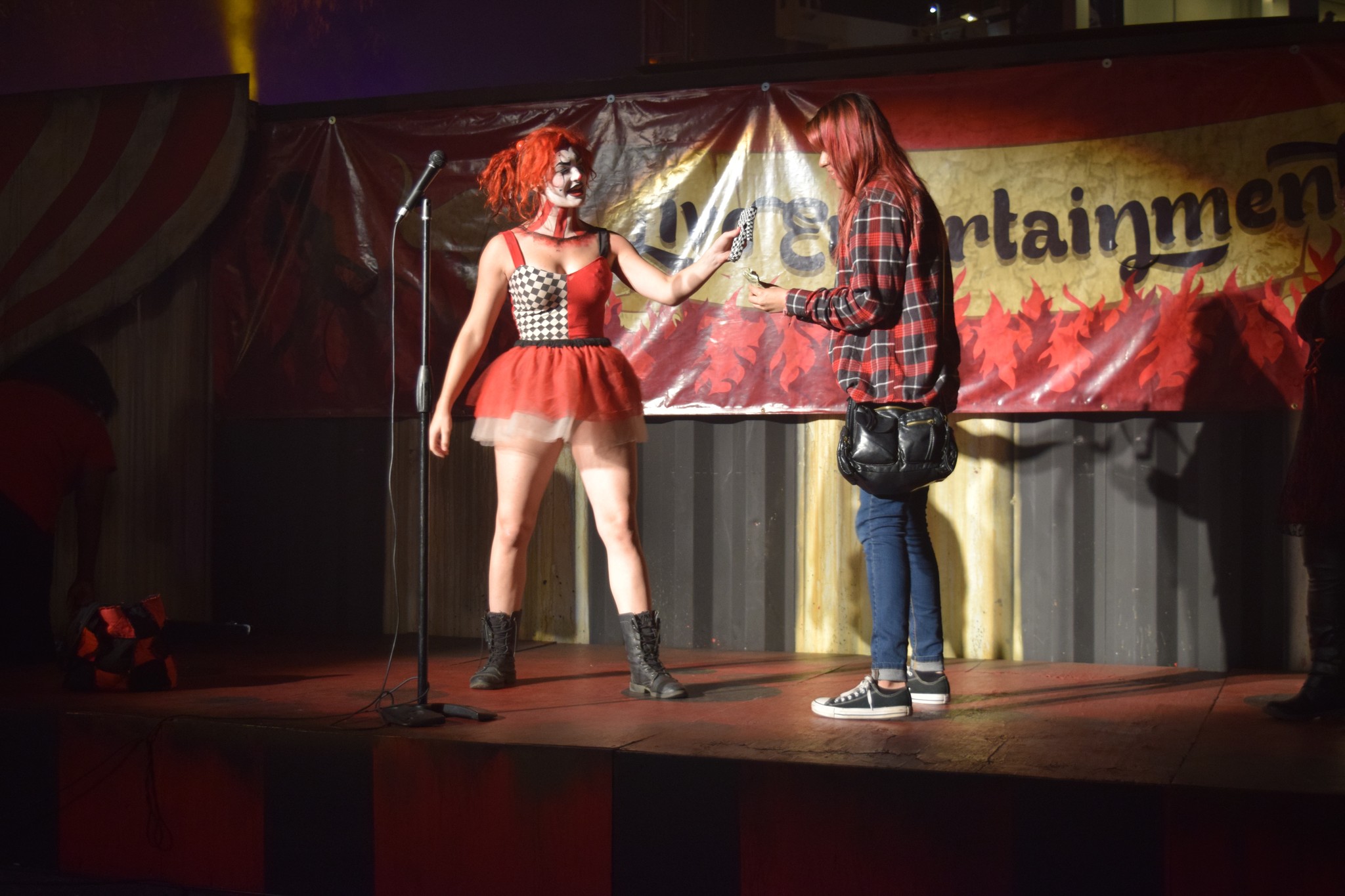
[381,703,445,727]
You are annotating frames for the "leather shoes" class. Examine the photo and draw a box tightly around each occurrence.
[1263,677,1344,722]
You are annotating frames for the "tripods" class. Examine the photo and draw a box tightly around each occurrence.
[363,198,500,725]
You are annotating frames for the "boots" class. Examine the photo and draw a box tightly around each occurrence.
[618,611,687,699]
[470,610,522,688]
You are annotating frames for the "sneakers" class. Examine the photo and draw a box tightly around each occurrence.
[812,679,914,719]
[908,671,950,704]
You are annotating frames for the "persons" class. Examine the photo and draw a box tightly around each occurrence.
[7,344,123,679]
[420,122,758,707]
[740,91,964,721]
[1252,250,1345,733]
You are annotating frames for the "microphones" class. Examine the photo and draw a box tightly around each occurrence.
[395,150,448,223]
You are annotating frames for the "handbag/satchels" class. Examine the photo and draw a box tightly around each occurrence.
[68,595,180,692]
[838,404,960,499]
